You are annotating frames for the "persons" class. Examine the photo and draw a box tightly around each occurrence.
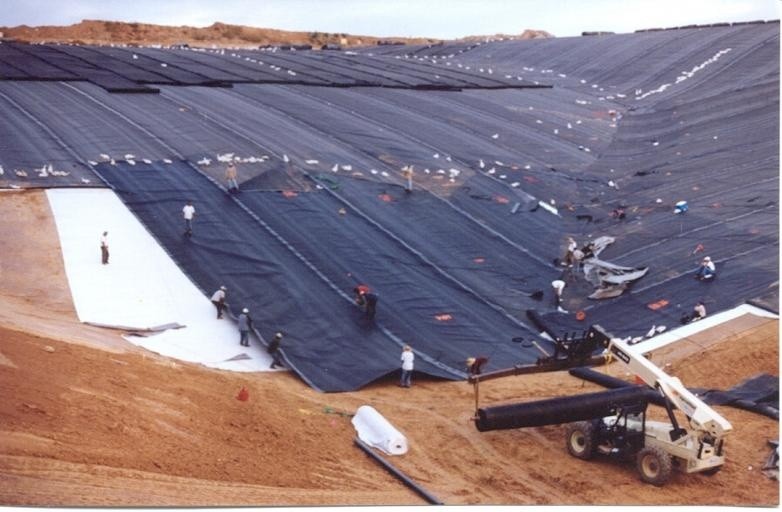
[353,284,371,311]
[100,229,109,266]
[224,160,240,195]
[399,165,413,193]
[360,289,379,319]
[180,199,195,237]
[400,345,415,389]
[237,306,253,348]
[693,255,716,282]
[551,280,568,303]
[466,357,486,375]
[211,285,229,320]
[561,236,578,269]
[690,298,706,323]
[267,332,284,371]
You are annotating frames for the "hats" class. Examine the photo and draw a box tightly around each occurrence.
[704,256,711,261]
[243,308,249,313]
[221,286,227,290]
[403,345,412,352]
[467,357,476,367]
[277,333,283,338]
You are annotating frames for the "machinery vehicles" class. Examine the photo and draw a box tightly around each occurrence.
[554,323,732,487]
